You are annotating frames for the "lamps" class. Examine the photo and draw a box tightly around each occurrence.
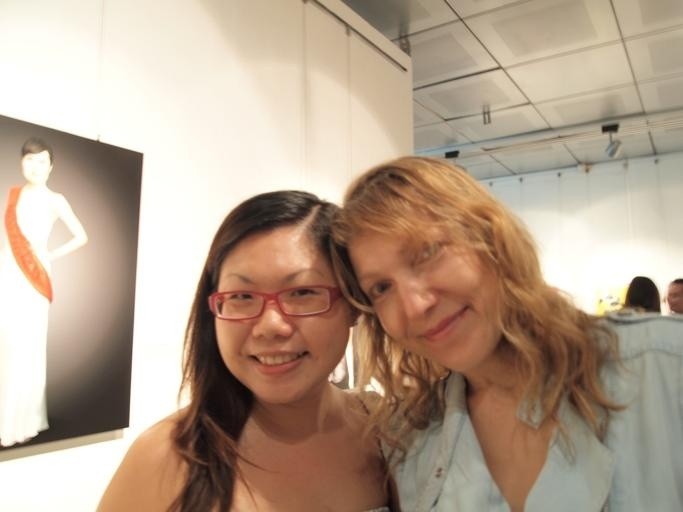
[600,123,622,159]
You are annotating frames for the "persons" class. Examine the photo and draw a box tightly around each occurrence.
[618,276,661,313]
[0,138,89,447]
[97,189,400,512]
[667,279,683,317]
[327,154,683,512]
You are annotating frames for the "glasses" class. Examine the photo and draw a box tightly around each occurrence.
[208,285,342,320]
